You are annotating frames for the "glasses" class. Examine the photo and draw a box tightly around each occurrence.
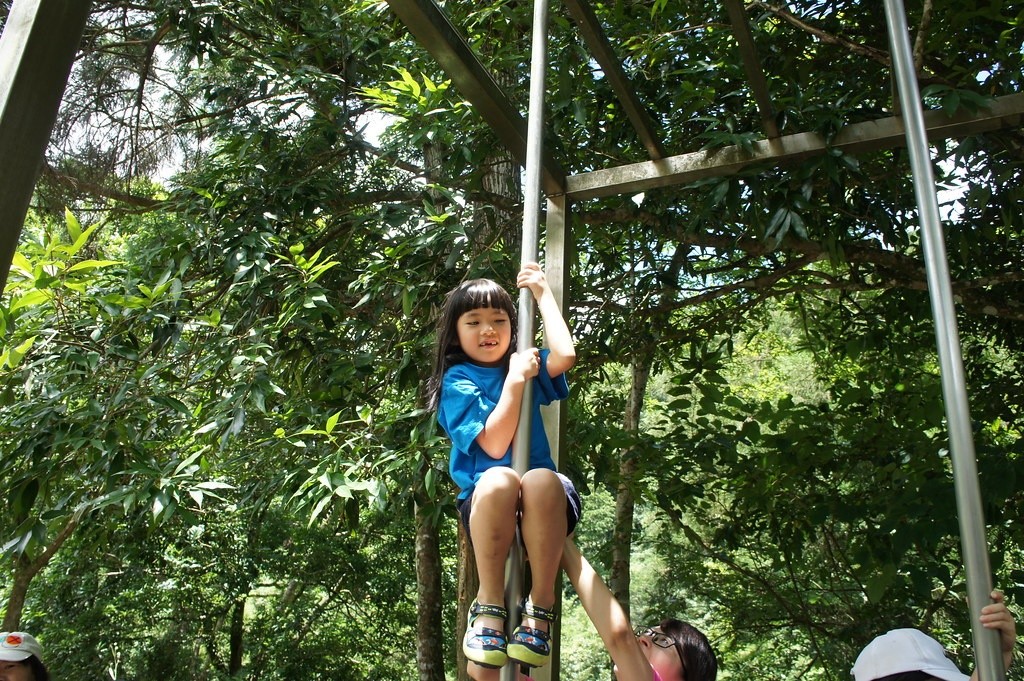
[634,625,688,673]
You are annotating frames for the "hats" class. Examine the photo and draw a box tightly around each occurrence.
[0,631,42,661]
[851,628,971,681]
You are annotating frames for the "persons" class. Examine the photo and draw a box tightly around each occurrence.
[465,537,717,681]
[0,631,50,681]
[432,262,580,669]
[854,590,1016,681]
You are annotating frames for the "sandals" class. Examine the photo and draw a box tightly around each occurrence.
[461,596,509,668]
[507,596,558,667]
